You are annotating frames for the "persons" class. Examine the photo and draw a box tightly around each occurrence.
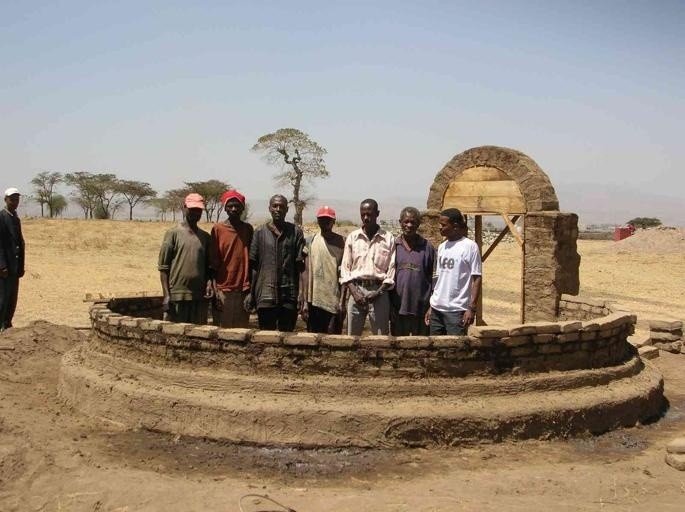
[301,204,347,334]
[156,191,220,322]
[247,191,305,332]
[391,204,436,335]
[424,206,484,335]
[339,198,397,336]
[0,187,26,331]
[210,187,254,327]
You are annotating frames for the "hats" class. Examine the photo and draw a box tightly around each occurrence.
[317,206,337,220]
[222,191,245,212]
[185,193,205,210]
[5,187,21,197]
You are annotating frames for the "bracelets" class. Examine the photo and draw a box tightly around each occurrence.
[466,303,479,313]
[376,288,384,297]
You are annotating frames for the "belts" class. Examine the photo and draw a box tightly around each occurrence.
[354,279,381,286]
